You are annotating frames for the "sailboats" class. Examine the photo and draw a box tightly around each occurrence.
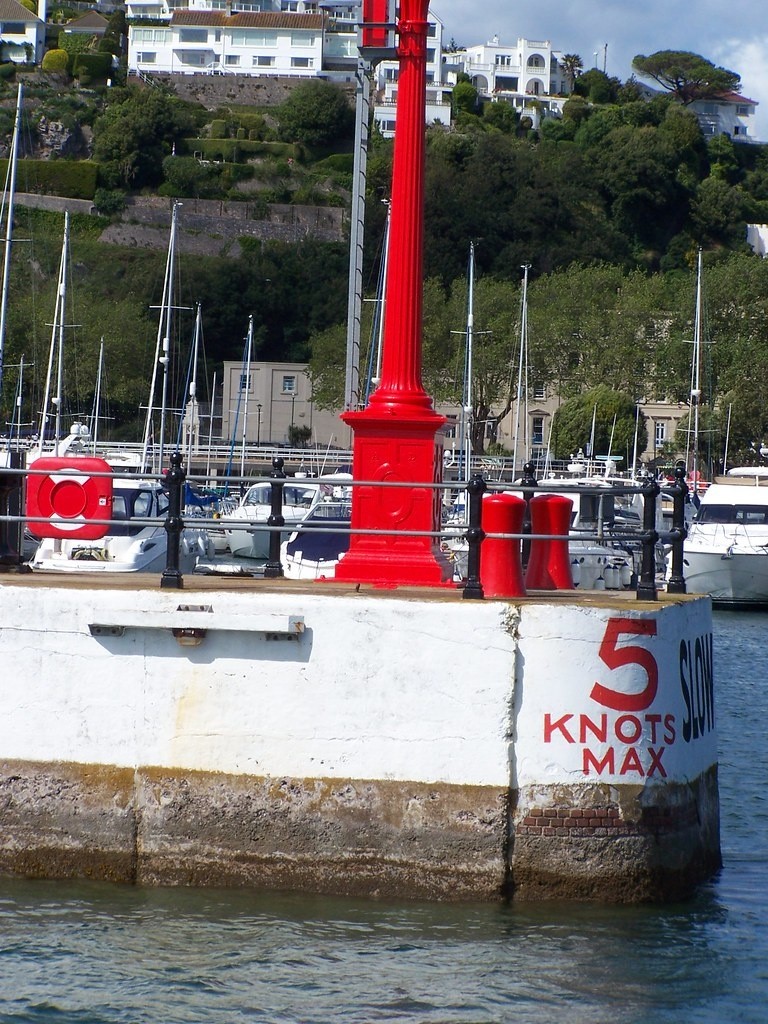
[0,82,768,603]
